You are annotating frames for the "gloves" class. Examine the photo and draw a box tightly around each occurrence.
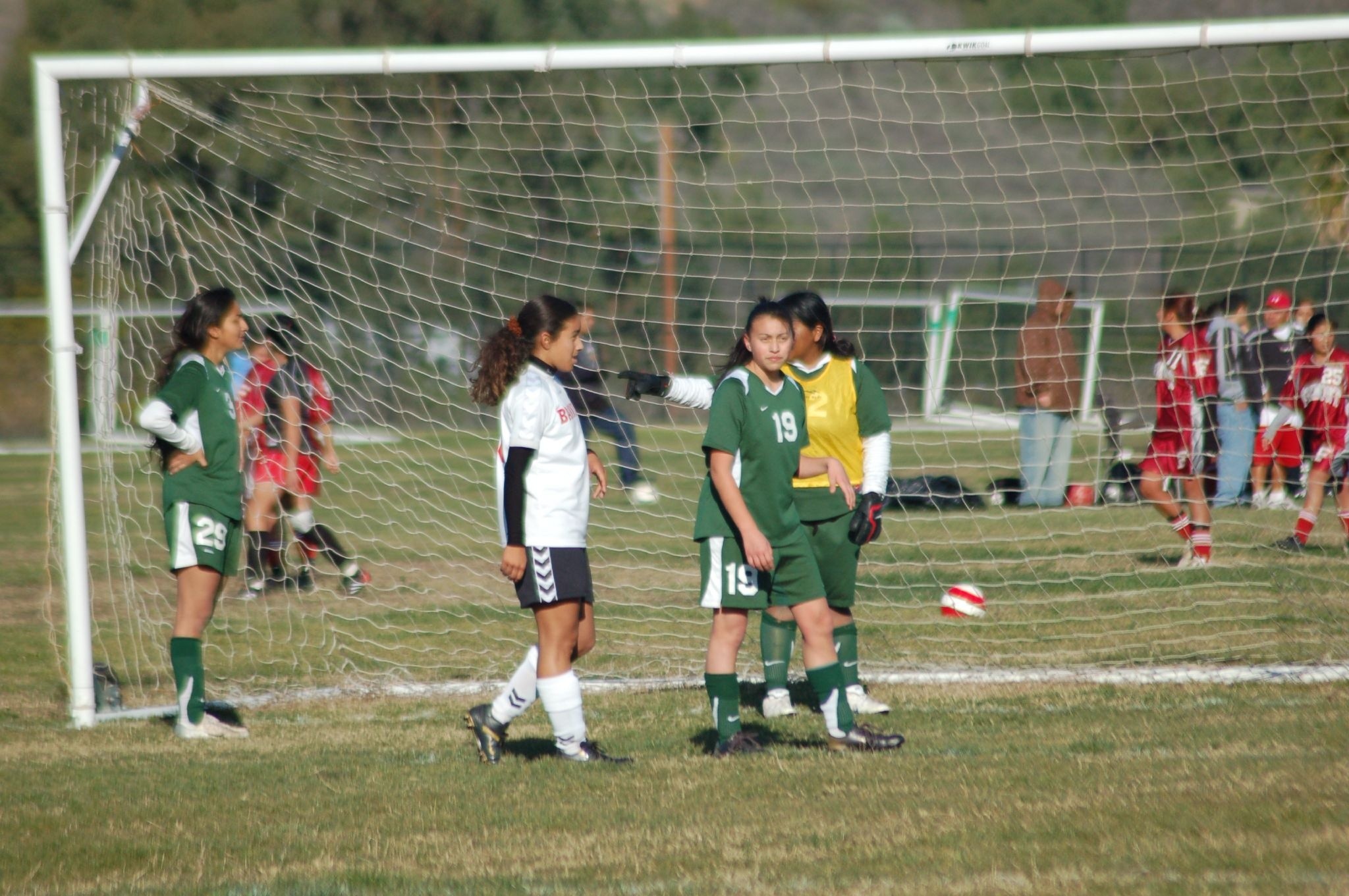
[848,491,886,544]
[616,371,667,403]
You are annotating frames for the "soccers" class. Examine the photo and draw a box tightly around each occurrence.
[940,582,987,620]
[1063,484,1094,507]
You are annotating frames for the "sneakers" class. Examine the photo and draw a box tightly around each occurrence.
[463,701,507,764]
[234,579,267,602]
[828,726,904,751]
[342,571,363,599]
[713,732,764,757]
[548,737,632,764]
[760,694,797,720]
[299,569,316,592]
[847,685,892,715]
[174,714,248,740]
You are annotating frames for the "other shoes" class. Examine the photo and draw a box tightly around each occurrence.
[1178,540,1208,573]
[1270,540,1302,552]
[1266,497,1299,512]
[1251,497,1268,513]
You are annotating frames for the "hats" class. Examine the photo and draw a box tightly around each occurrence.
[1265,292,1290,311]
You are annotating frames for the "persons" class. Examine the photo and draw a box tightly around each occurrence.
[692,293,904,759]
[556,300,662,505]
[469,295,634,768]
[1262,316,1349,556]
[140,287,249,741]
[229,312,370,600]
[1138,289,1213,571]
[1194,290,1344,512]
[618,292,891,722]
[1016,278,1077,510]
